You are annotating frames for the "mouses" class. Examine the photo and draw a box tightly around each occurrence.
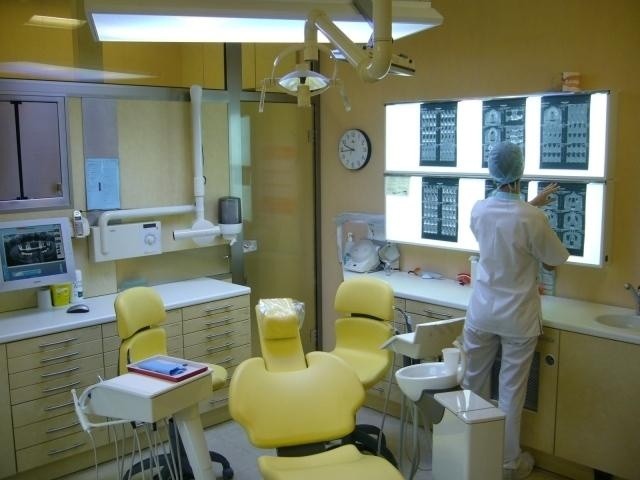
[66,303,90,313]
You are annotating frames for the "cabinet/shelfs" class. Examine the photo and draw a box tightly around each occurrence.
[554,324,640,480]
[101,308,185,461]
[182,293,253,430]
[5,322,108,478]
[343,294,466,433]
[490,325,555,476]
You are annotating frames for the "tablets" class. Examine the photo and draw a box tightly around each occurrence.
[125,354,207,383]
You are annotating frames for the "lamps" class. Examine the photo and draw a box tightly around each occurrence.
[255,1,394,113]
[82,0,446,47]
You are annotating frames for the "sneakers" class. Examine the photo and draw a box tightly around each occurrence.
[502,451,535,480]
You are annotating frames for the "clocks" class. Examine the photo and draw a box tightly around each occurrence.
[338,127,371,171]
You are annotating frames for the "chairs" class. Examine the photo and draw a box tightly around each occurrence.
[373,315,467,479]
[113,285,235,479]
[226,297,407,480]
[327,275,399,469]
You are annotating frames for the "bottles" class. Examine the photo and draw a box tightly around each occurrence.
[343,232,355,265]
[72,269,84,303]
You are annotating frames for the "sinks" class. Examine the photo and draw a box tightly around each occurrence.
[593,312,640,333]
[395,361,461,402]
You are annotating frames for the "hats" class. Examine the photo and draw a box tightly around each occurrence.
[487,141,526,186]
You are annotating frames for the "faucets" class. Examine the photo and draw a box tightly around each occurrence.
[624,283,640,317]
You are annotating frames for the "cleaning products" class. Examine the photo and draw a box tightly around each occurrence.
[53,270,84,305]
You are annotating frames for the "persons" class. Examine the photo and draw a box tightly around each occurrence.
[462,141,570,480]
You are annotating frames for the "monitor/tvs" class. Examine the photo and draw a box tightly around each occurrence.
[0,216,77,294]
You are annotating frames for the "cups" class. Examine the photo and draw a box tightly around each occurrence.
[36,289,52,311]
[441,347,461,375]
